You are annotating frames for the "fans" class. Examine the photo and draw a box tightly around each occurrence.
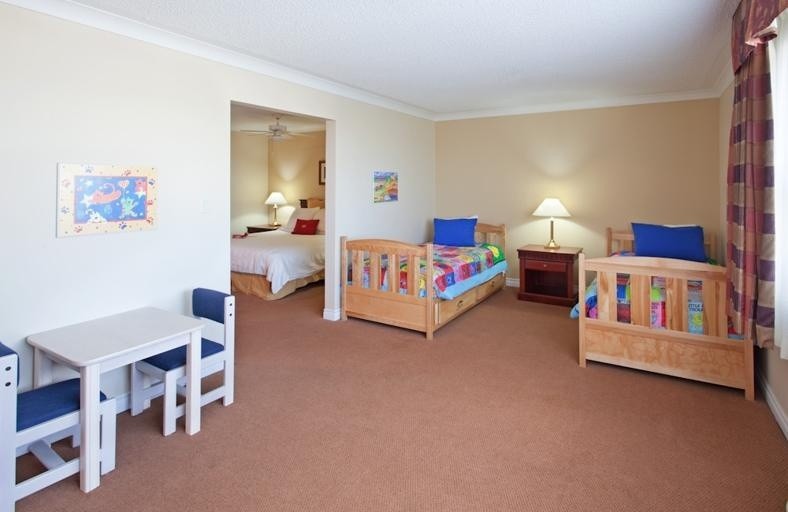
[236,113,315,142]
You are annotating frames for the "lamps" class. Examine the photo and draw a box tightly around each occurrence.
[264,191,289,227]
[530,195,572,249]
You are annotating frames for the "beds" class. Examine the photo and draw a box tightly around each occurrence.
[576,222,758,403]
[229,196,326,303]
[339,217,509,343]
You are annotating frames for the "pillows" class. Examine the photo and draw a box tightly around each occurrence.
[431,216,478,246]
[430,210,479,231]
[278,206,321,234]
[288,217,321,235]
[310,207,326,234]
[629,222,710,264]
[662,222,699,231]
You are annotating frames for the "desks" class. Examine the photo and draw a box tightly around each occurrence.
[28,303,209,496]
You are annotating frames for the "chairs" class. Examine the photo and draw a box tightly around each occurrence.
[125,286,237,439]
[0,339,117,512]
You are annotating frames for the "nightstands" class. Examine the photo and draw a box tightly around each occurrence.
[245,223,281,234]
[516,243,585,309]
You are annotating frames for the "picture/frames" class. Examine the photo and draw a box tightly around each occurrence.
[318,160,327,186]
[53,162,160,240]
